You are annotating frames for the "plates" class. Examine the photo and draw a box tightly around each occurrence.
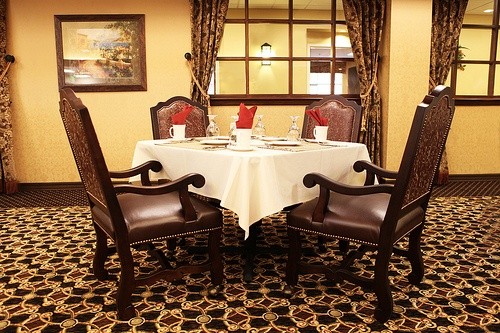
[226,145,256,151]
[196,136,229,140]
[200,140,229,144]
[306,138,331,143]
[265,141,304,145]
[167,138,191,140]
[261,137,286,141]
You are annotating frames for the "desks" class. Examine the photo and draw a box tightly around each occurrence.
[129,136,379,282]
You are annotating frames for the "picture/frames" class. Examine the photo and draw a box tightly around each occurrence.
[53,14,147,92]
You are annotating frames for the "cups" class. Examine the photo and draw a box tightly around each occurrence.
[313,126,329,141]
[169,125,186,139]
[229,129,252,148]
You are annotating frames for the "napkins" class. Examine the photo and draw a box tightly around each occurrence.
[169,105,194,124]
[305,108,329,126]
[236,103,258,129]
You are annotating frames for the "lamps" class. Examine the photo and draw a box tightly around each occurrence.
[261,42,272,66]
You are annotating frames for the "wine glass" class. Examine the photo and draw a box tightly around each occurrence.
[230,116,239,130]
[206,115,220,137]
[252,115,266,136]
[287,116,301,142]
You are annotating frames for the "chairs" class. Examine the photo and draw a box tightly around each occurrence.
[287,84,455,324]
[150,95,209,140]
[59,88,223,321]
[301,96,362,142]
